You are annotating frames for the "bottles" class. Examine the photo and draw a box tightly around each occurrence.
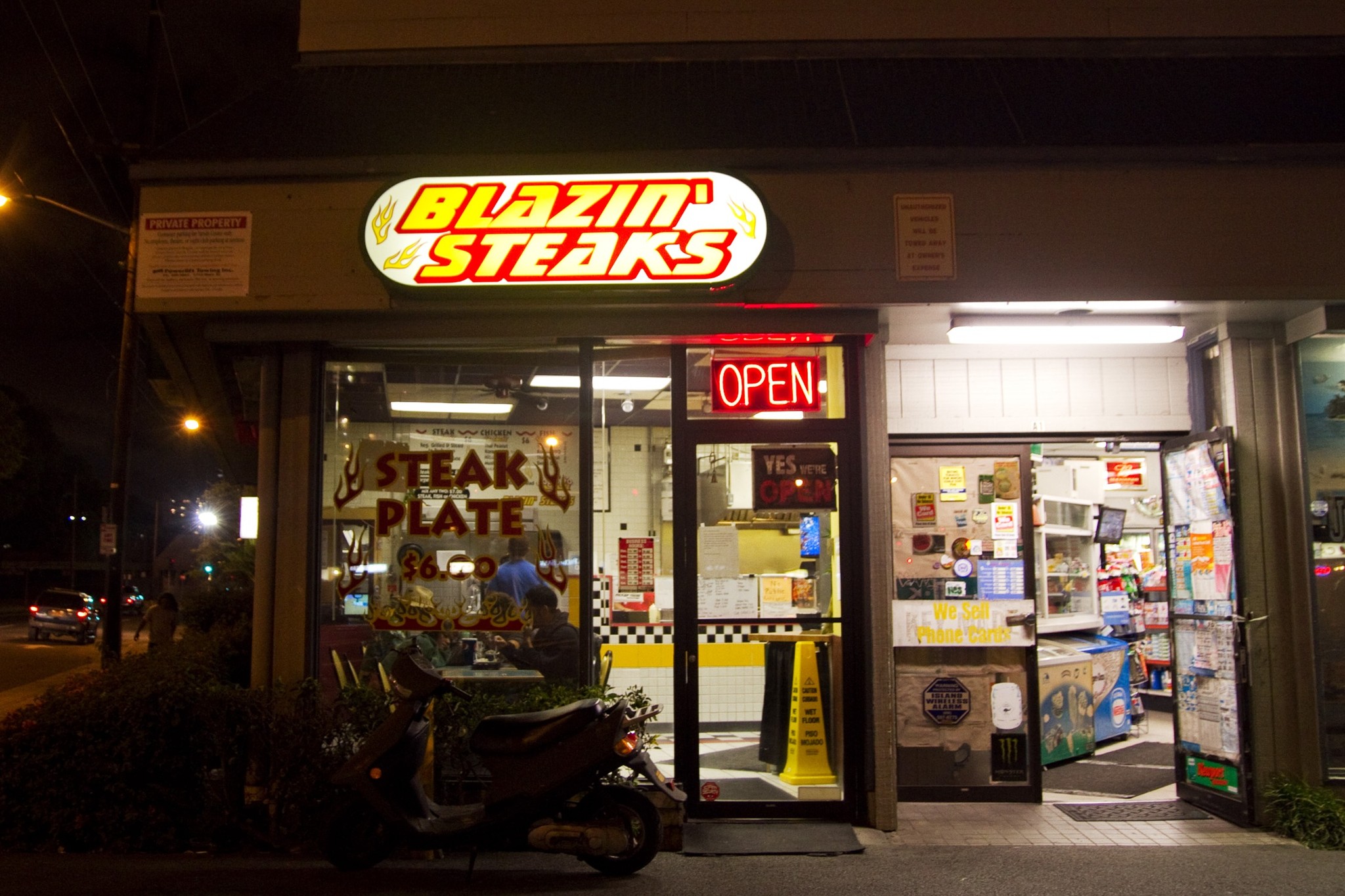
[1150,666,1173,693]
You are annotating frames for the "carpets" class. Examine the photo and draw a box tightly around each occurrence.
[1093,740,1174,766]
[680,821,868,857]
[1052,801,1216,822]
[704,779,795,799]
[655,743,767,772]
[1043,761,1176,797]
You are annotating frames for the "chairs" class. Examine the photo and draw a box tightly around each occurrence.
[599,649,612,692]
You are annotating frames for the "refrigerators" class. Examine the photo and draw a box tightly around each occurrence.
[1037,632,1132,772]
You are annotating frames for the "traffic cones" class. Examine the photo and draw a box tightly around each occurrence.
[778,640,839,786]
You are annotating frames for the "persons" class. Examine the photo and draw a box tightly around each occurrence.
[359,584,463,768]
[484,536,547,619]
[480,584,580,692]
[134,591,181,652]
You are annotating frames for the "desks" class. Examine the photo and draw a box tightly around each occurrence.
[439,662,547,692]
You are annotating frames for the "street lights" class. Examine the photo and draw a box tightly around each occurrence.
[0,185,142,661]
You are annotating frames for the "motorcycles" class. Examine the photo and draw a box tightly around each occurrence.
[332,635,688,878]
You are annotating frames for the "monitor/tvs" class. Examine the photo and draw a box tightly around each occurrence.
[800,512,820,558]
[1093,506,1127,545]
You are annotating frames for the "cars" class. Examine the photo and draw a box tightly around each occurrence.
[29,589,101,647]
[101,584,146,610]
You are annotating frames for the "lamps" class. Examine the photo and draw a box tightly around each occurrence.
[620,394,635,413]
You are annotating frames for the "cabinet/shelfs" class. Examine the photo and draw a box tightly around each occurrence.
[1033,492,1099,633]
[1139,588,1171,695]
